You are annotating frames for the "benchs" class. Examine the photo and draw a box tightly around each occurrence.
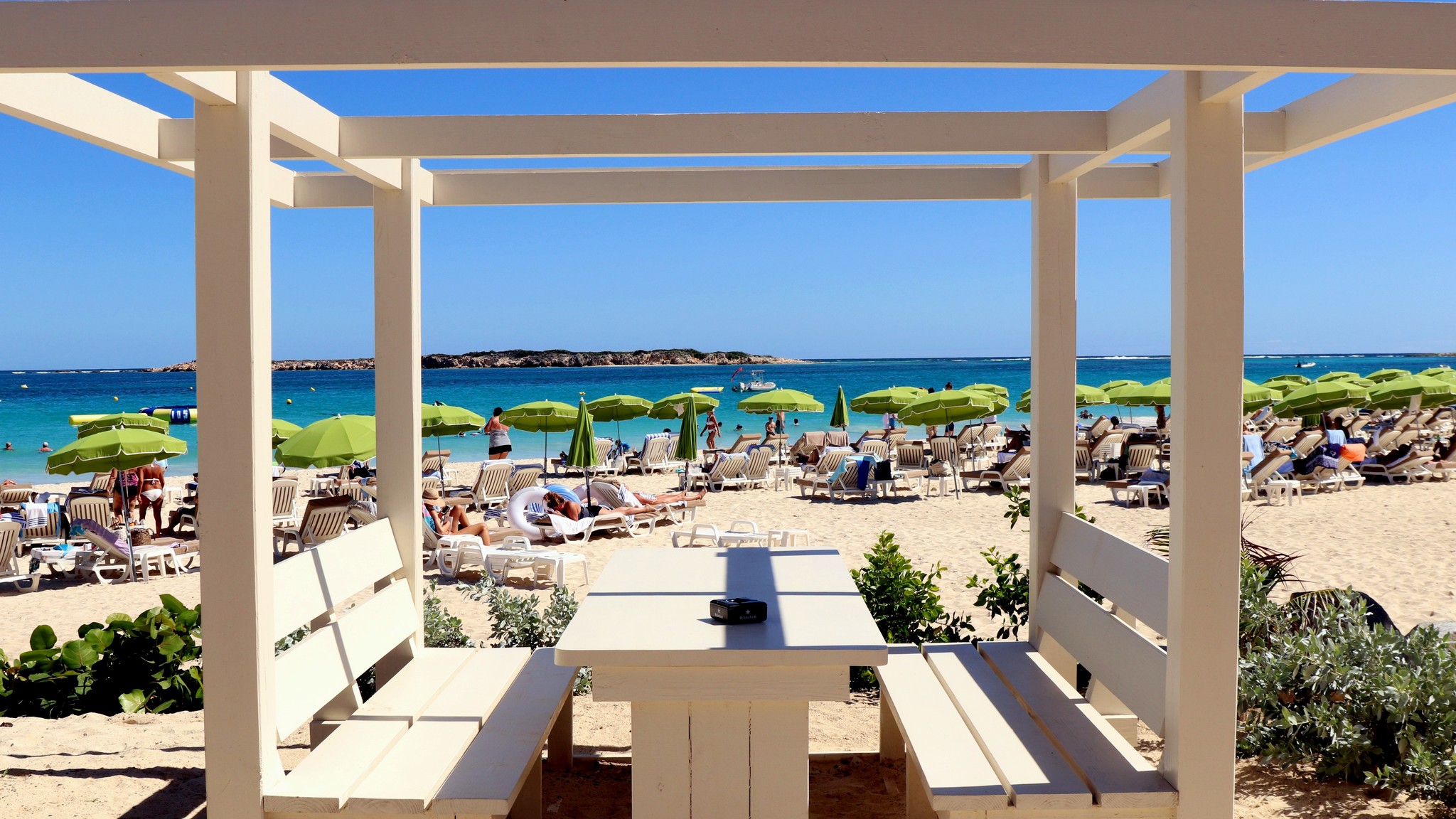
[270,518,580,819]
[871,513,1168,819]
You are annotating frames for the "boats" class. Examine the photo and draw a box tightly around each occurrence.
[139,405,198,425]
[690,387,724,393]
[731,370,776,393]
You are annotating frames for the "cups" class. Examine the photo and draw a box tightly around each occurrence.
[128,518,132,523]
[975,456,980,459]
[966,450,971,458]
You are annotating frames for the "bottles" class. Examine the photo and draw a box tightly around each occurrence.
[1437,434,1439,440]
[129,522,135,526]
[1104,453,1107,462]
[83,543,88,551]
[1001,430,1003,437]
[91,543,96,551]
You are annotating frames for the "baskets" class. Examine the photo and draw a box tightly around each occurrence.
[130,528,151,546]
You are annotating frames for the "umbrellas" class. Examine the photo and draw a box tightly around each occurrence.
[500,383,1009,500]
[421,403,486,499]
[566,396,598,505]
[45,423,188,575]
[274,414,377,497]
[272,419,303,450]
[674,393,697,520]
[76,411,169,509]
[1016,371,1456,430]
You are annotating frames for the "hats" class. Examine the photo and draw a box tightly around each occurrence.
[769,416,773,419]
[422,488,445,507]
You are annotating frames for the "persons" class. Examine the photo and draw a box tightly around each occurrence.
[106,459,168,538]
[484,406,1121,518]
[1263,439,1295,450]
[38,442,52,451]
[422,488,490,546]
[6,442,12,450]
[998,424,1030,453]
[1155,405,1166,429]
[1433,432,1456,460]
[336,460,377,486]
[160,492,198,535]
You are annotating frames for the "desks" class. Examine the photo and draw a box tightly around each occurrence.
[1267,480,1303,507]
[131,546,182,583]
[445,468,459,486]
[768,529,810,549]
[678,472,708,492]
[76,550,109,579]
[534,552,589,589]
[554,547,890,819]
[1127,485,1162,507]
[310,477,336,497]
[926,443,1122,497]
[499,510,528,528]
[163,486,184,504]
[775,467,804,492]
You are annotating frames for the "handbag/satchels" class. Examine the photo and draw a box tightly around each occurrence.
[105,517,127,543]
[1100,468,1115,480]
[928,460,952,477]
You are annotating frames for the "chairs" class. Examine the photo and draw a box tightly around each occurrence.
[1242,404,1456,501]
[760,433,789,465]
[0,405,781,592]
[1076,416,1170,506]
[789,416,1031,503]
